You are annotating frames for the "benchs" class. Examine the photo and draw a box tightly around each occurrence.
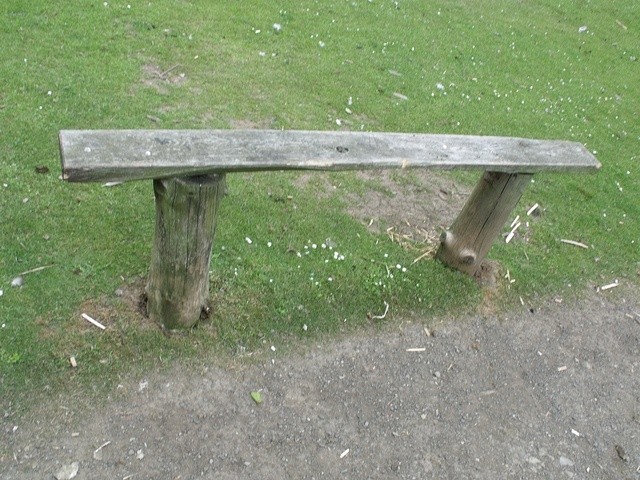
[58,127,602,330]
